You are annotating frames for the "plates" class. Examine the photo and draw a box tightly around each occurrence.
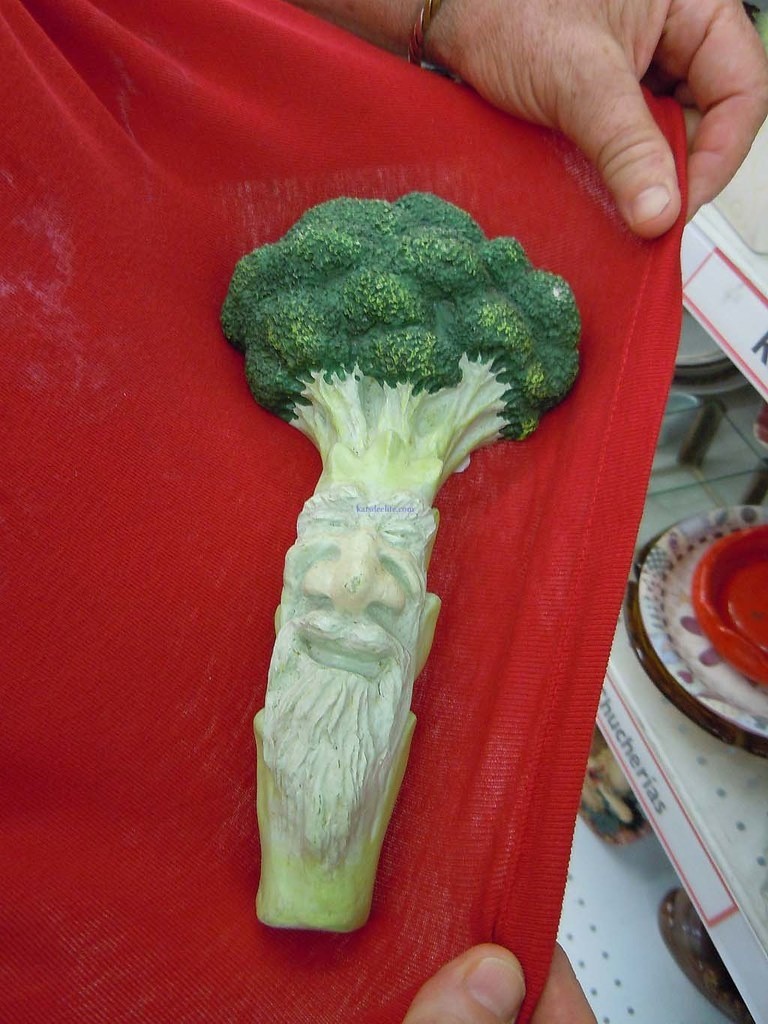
[674,304,752,402]
[624,504,768,758]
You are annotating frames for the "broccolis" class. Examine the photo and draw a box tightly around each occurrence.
[220,190,582,934]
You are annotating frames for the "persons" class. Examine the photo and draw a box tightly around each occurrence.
[401,941,600,1024]
[271,0,765,234]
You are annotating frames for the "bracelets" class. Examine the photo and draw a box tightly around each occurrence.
[407,0,441,69]
